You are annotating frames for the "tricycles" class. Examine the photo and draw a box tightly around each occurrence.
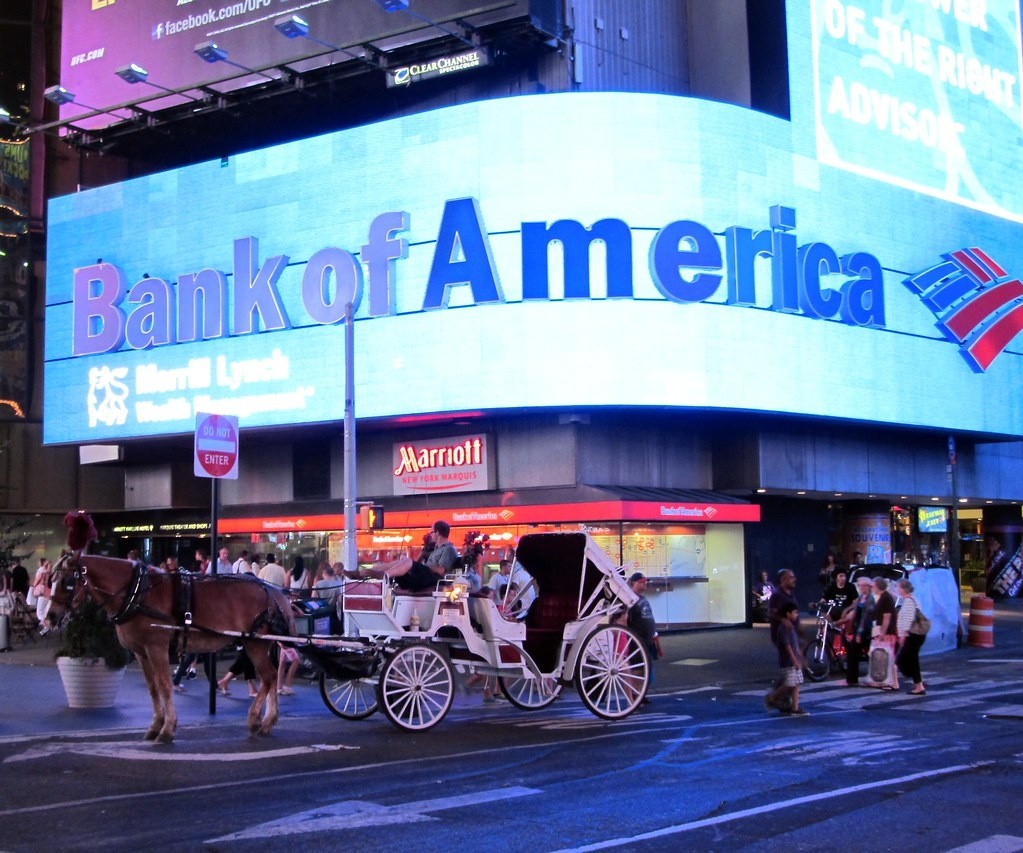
[803,601,868,682]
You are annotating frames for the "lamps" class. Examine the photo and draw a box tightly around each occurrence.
[449,573,468,604]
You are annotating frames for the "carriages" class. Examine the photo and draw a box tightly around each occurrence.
[28,530,652,742]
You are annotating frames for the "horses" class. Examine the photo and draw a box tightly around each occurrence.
[43,555,298,743]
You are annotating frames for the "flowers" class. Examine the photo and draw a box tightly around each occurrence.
[422,531,435,549]
[462,529,493,571]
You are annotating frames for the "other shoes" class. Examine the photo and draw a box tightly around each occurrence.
[172,667,186,677]
[215,687,231,696]
[188,672,197,680]
[249,692,258,697]
[493,692,506,698]
[482,697,504,705]
[276,685,294,694]
[341,569,384,581]
[907,688,926,695]
[459,683,471,699]
[634,695,651,704]
[764,696,810,716]
[39,628,50,635]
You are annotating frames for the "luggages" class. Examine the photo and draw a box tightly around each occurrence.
[0,605,10,653]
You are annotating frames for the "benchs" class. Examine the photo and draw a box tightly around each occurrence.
[470,595,526,641]
[523,592,591,652]
[391,573,469,627]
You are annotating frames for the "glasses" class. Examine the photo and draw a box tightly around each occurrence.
[429,530,437,535]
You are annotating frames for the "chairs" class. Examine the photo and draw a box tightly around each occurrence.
[7,592,40,644]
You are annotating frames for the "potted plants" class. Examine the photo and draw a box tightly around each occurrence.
[965,561,987,591]
[50,598,135,710]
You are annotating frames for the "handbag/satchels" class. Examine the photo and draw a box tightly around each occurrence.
[864,635,899,689]
[34,572,46,596]
[25,586,38,605]
[908,597,930,635]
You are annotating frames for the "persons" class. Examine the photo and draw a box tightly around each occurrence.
[627,571,664,705]
[231,549,252,574]
[751,571,776,623]
[341,520,456,592]
[609,610,640,705]
[457,547,554,701]
[256,552,286,586]
[852,550,864,564]
[894,580,927,695]
[0,546,232,654]
[217,646,259,698]
[283,555,310,591]
[764,602,811,716]
[250,554,261,576]
[768,568,797,714]
[310,562,349,635]
[816,551,842,597]
[172,652,220,693]
[819,569,898,716]
[277,588,305,695]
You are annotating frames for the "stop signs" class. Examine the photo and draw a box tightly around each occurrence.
[194,411,238,480]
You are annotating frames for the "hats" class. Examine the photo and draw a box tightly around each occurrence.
[857,577,874,587]
[630,572,650,583]
